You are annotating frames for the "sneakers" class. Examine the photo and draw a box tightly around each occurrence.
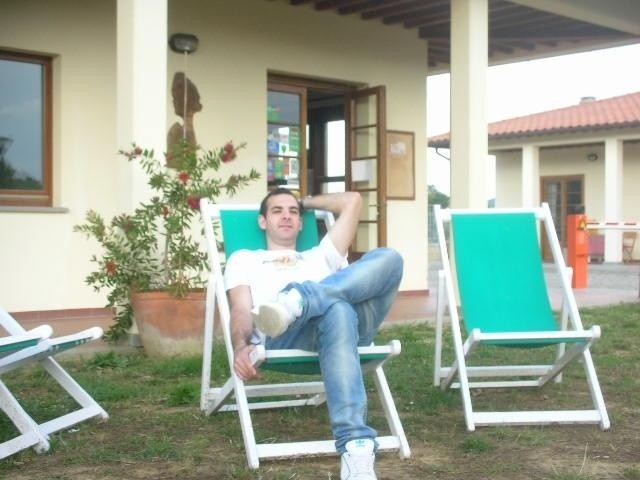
[250,288,304,339]
[340,438,378,479]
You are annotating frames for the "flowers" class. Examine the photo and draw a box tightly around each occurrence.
[68,136,266,363]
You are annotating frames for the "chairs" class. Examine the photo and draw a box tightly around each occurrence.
[433,201,611,432]
[1,293,110,462]
[200,197,412,468]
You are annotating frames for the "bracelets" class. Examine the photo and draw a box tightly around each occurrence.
[298,195,313,211]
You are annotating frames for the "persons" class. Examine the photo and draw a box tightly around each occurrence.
[221,188,406,480]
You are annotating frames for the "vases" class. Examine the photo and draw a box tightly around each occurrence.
[129,290,220,365]
